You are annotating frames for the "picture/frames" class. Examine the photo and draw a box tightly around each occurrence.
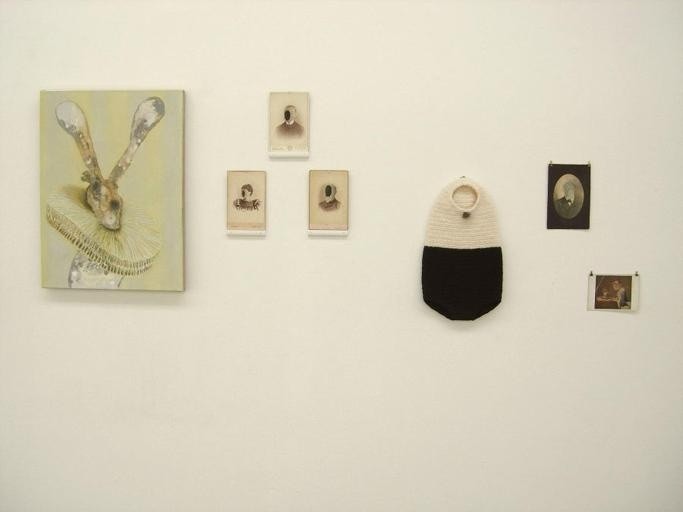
[586,273,639,313]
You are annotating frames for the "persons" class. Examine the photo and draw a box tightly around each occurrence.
[232,184,261,211]
[274,105,304,140]
[318,183,343,212]
